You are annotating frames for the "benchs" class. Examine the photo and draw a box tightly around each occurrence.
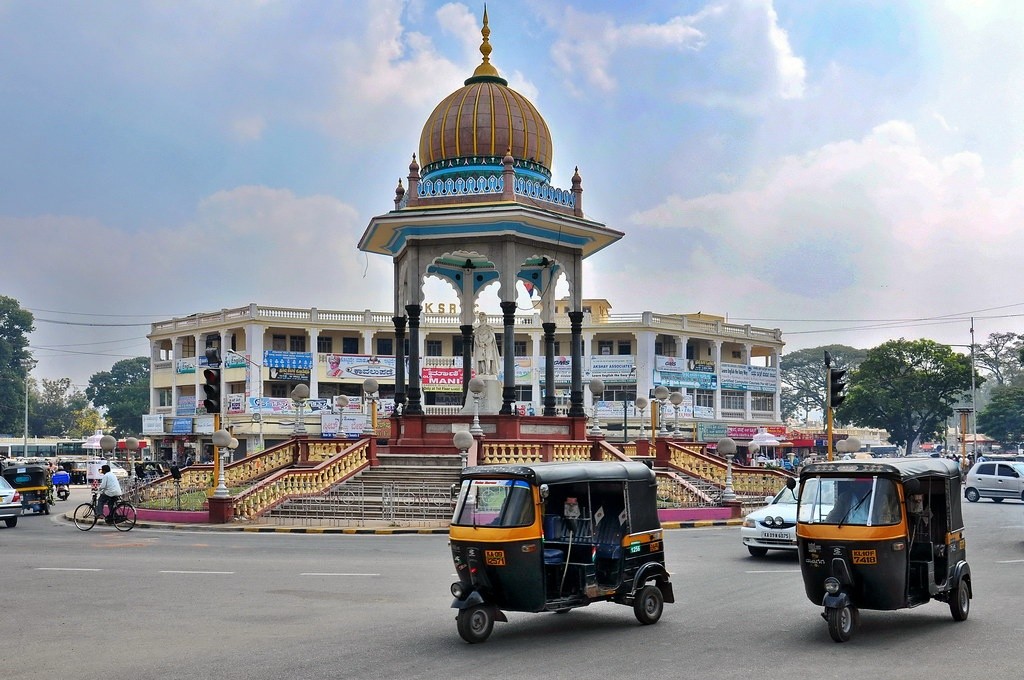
[542,516,622,561]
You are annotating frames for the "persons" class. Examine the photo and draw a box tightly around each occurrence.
[941,451,988,480]
[823,480,891,524]
[48,451,214,526]
[733,454,829,477]
[487,487,546,527]
[472,313,496,375]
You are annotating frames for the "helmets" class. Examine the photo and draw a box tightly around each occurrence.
[58,466,64,469]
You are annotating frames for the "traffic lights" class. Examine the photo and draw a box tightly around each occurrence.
[828,367,847,408]
[203,367,221,414]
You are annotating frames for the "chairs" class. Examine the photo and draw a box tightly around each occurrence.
[542,516,566,568]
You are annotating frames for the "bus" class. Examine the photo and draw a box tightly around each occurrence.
[54,435,88,485]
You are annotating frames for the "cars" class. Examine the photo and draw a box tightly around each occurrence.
[0,453,128,485]
[740,476,835,559]
[963,460,1024,504]
[0,476,23,528]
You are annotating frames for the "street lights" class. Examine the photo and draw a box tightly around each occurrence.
[624,365,637,443]
[227,348,264,451]
[933,344,977,464]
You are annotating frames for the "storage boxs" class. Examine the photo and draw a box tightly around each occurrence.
[52,470,72,487]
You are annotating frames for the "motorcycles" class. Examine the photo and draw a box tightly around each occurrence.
[785,455,973,644]
[445,460,676,645]
[1,460,55,515]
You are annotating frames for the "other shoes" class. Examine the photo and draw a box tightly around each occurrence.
[94,514,100,517]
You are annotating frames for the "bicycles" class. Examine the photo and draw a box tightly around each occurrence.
[73,487,137,532]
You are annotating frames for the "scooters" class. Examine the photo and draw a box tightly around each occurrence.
[55,472,70,501]
